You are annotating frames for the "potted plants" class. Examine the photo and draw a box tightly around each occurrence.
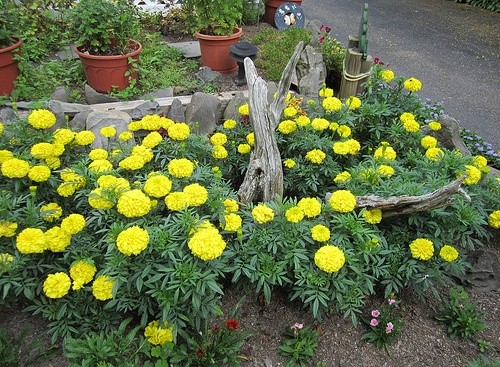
[66,0,142,95]
[182,1,243,75]
[0,2,25,98]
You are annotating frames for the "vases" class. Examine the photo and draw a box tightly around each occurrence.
[264,0,302,29]
